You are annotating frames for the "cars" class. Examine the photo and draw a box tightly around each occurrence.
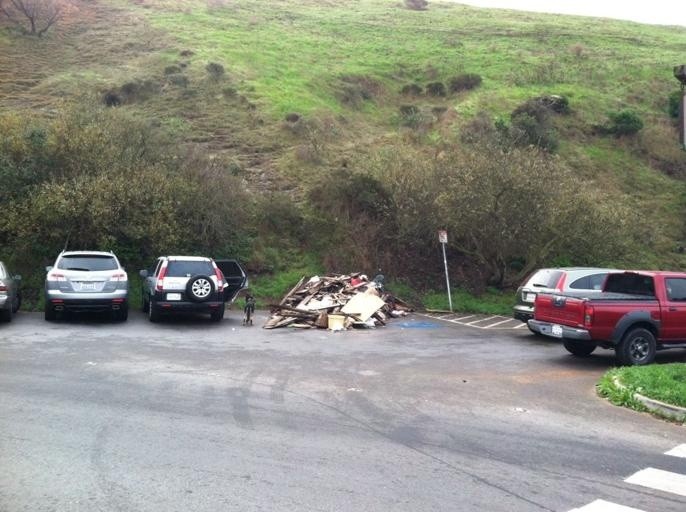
[0,261,22,323]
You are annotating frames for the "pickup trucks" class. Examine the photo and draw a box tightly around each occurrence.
[529,270,686,366]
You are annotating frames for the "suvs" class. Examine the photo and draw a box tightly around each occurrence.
[41,250,130,322]
[138,254,248,324]
[513,267,612,330]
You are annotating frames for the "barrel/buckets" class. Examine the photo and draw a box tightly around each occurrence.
[328,315,345,330]
